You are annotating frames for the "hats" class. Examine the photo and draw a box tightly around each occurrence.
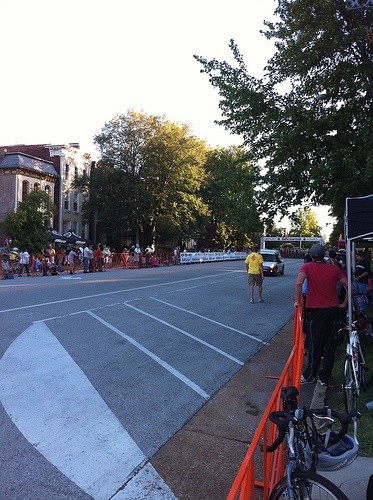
[336,249,346,255]
[356,250,365,256]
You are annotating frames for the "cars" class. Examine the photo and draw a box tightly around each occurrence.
[257,250,285,277]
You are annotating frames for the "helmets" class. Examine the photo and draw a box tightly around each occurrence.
[306,429,359,472]
[310,244,325,260]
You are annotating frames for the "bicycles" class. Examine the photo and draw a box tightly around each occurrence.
[337,315,365,418]
[264,385,371,499]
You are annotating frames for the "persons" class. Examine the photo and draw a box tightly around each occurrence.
[295,244,355,386]
[301,245,370,318]
[279,247,309,259]
[9,241,244,273]
[18,248,31,277]
[244,245,265,303]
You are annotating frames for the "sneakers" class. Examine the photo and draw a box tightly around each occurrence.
[250,300,254,304]
[316,371,329,385]
[300,375,316,385]
[258,298,265,303]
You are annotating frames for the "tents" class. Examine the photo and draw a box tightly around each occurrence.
[46,227,68,255]
[61,229,89,262]
